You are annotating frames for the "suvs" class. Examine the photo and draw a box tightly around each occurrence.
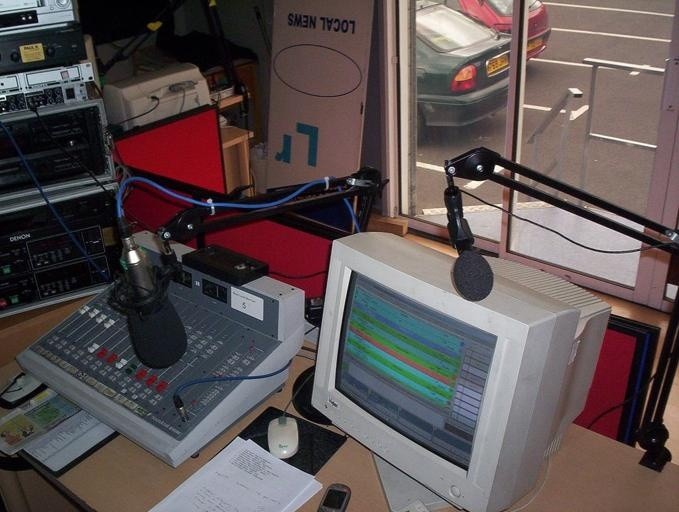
[432,0,552,61]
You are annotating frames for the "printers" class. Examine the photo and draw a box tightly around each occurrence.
[102,61,213,133]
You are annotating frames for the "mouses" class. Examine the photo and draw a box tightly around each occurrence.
[266,414,299,460]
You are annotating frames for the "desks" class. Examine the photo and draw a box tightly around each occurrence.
[132,48,261,144]
[1,293,679,510]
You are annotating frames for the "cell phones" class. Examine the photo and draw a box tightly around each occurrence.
[316,482,352,512]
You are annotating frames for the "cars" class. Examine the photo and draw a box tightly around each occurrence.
[415,0,512,144]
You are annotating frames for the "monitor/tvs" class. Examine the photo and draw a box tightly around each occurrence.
[311,231,613,512]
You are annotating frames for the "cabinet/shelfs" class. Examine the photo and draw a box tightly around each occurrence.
[222,125,255,197]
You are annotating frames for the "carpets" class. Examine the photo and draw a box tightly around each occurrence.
[170,208,661,448]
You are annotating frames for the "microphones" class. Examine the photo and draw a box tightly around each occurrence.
[451,242,495,301]
[116,222,189,370]
